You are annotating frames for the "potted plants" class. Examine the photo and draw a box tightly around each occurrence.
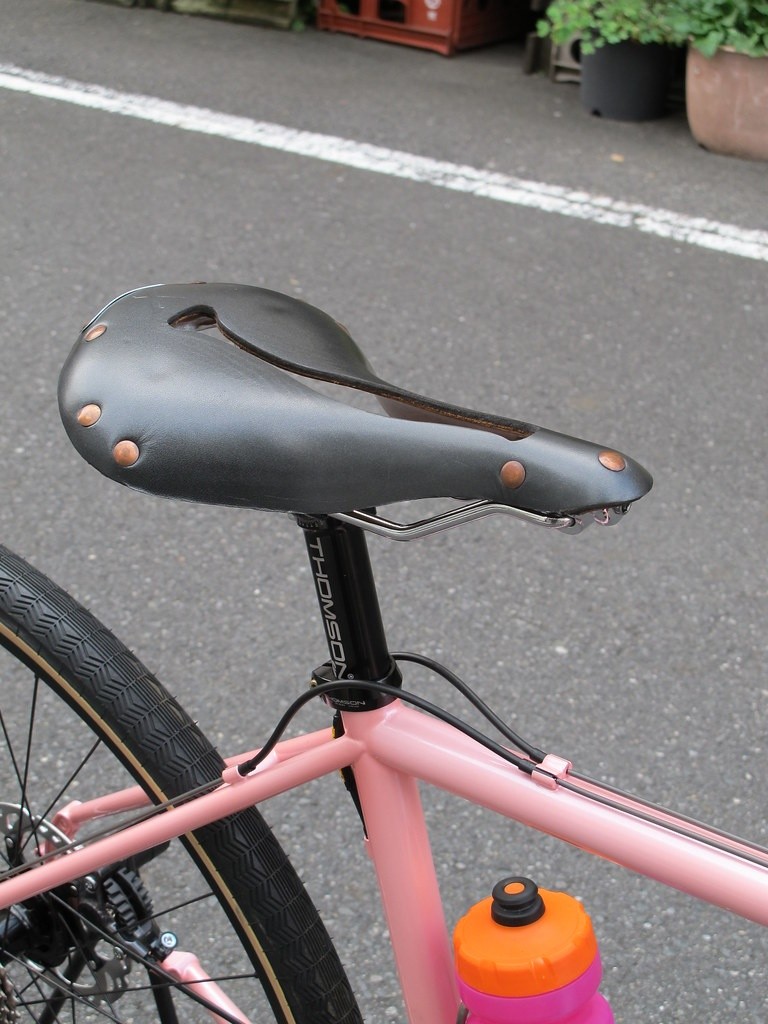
[536,0,689,122]
[685,0,768,162]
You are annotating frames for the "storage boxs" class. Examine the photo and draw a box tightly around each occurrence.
[316,0,530,55]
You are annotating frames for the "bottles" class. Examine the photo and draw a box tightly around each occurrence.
[451,875,615,1024]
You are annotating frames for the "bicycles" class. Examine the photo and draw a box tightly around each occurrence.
[0,283,768,1024]
